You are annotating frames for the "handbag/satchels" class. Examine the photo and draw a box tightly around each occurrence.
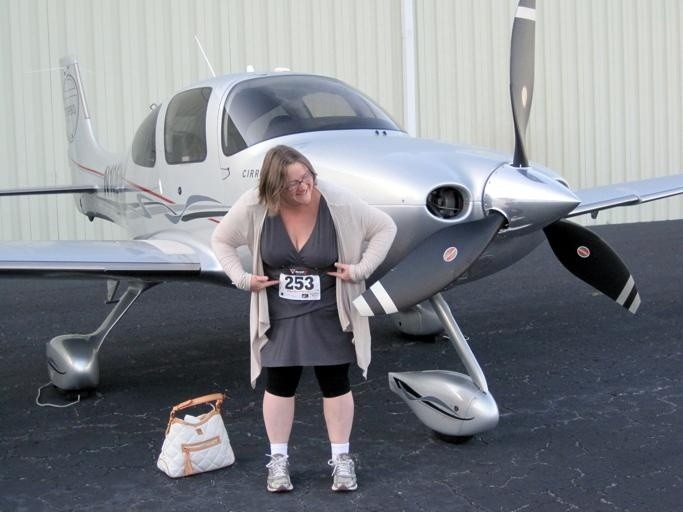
[157,393,234,479]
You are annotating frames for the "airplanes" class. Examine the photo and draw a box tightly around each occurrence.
[0,0,683,443]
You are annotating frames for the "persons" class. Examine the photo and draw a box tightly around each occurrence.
[212,144,398,495]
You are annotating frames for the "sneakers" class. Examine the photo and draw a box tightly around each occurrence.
[265,452,295,492]
[328,454,358,493]
